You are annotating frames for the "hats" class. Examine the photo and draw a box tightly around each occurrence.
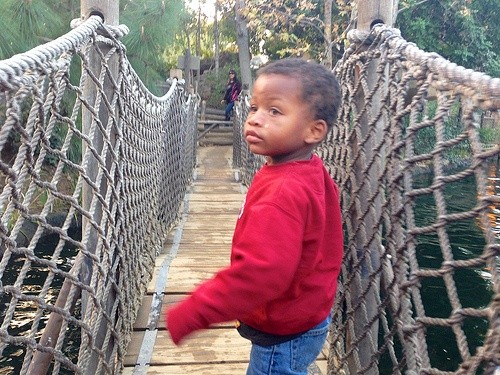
[229,70,236,74]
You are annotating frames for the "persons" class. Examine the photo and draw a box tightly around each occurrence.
[221,69,241,127]
[167,59,343,375]
